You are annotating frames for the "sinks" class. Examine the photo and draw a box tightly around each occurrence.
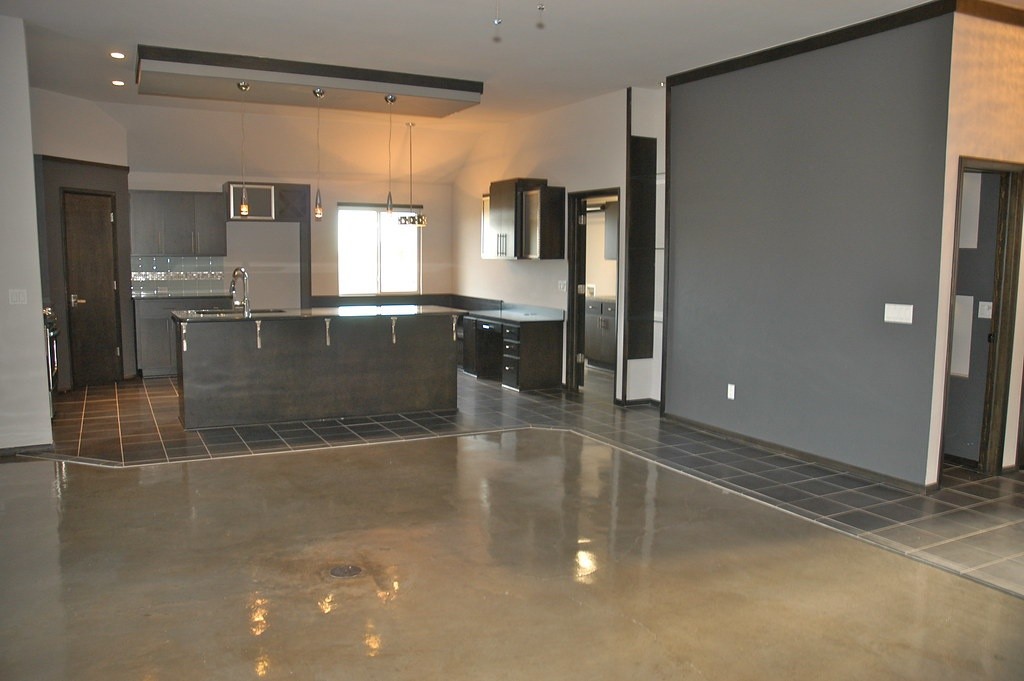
[195,308,286,315]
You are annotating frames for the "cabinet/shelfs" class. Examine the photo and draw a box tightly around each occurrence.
[126,189,228,257]
[481,178,566,261]
[585,200,617,369]
[462,315,521,393]
[135,299,233,378]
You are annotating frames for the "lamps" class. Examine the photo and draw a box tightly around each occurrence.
[385,94,396,210]
[397,122,426,225]
[238,82,249,216]
[313,88,323,218]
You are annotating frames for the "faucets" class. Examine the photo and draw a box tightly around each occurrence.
[228,266,252,319]
